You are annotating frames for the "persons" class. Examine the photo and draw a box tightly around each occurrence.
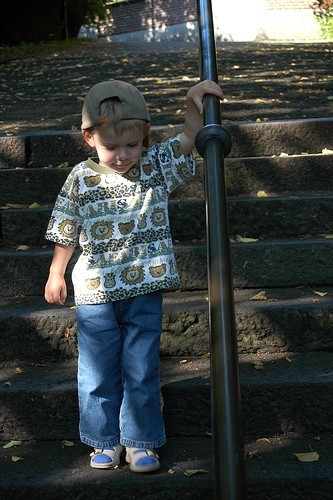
[43,78,224,472]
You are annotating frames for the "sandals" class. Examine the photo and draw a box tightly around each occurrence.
[89,443,124,470]
[122,447,161,473]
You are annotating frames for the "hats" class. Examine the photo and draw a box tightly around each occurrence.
[80,77,150,131]
